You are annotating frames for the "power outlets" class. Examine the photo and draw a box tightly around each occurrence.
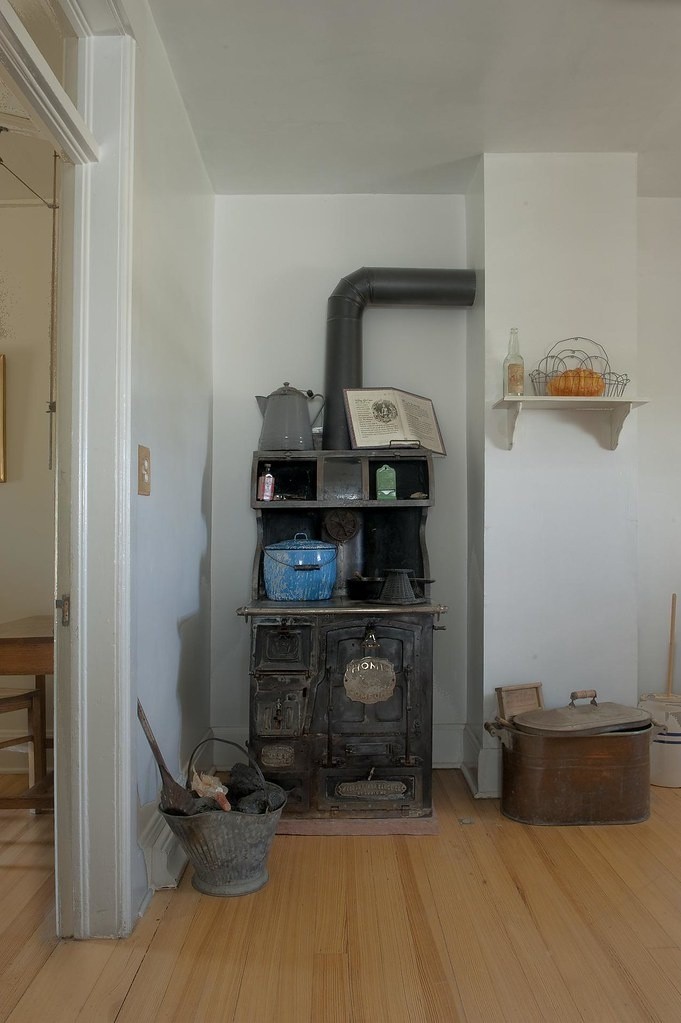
[138,444,151,495]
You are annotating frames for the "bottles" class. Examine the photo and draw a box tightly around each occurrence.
[257,463,276,501]
[503,328,525,397]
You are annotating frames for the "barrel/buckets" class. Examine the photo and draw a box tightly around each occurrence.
[264,532,337,601]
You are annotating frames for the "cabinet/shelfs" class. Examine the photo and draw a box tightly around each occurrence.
[234,450,447,837]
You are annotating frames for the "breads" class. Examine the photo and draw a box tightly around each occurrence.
[546,368,604,397]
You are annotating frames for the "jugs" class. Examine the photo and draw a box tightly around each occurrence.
[255,382,325,451]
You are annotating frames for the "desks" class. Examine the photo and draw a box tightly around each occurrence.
[0,615,55,816]
